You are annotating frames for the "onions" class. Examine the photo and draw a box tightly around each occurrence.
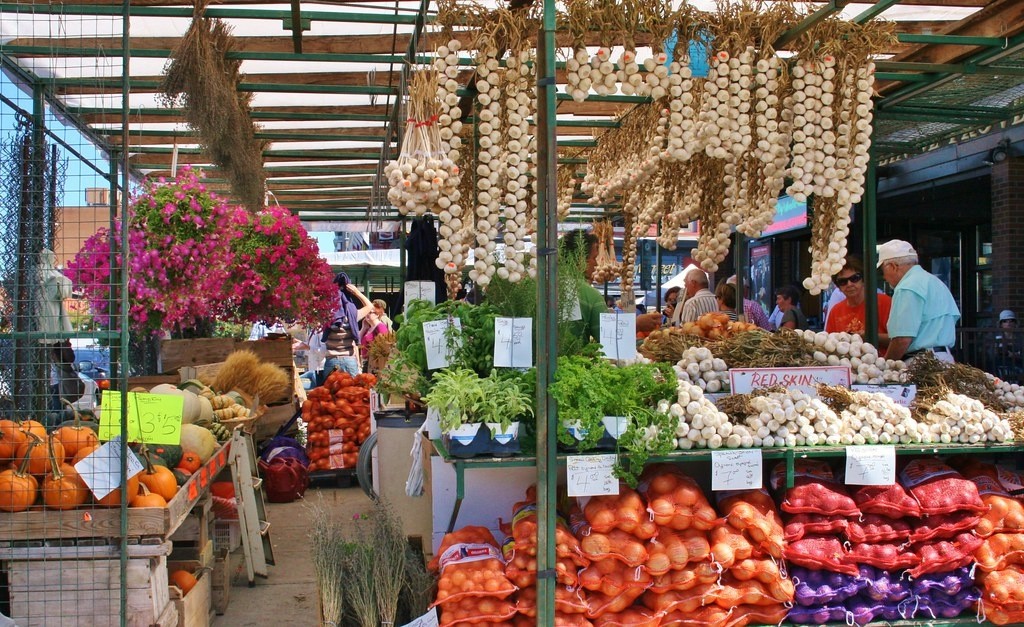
[303,372,377,471]
[640,312,758,349]
[426,458,1024,627]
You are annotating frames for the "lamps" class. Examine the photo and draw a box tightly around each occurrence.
[983,147,1007,166]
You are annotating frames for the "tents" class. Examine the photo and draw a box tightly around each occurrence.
[636,263,708,312]
[318,242,538,331]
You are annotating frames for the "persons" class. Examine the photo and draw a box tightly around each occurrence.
[558,229,609,352]
[303,274,394,417]
[0,316,98,424]
[248,314,303,410]
[877,240,960,365]
[663,268,808,333]
[824,256,892,357]
[605,296,645,320]
[456,288,482,307]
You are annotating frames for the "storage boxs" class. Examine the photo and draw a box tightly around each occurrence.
[167,520,242,627]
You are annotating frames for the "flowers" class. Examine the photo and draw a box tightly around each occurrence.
[56,161,341,343]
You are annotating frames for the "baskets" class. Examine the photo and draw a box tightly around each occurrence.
[212,519,241,553]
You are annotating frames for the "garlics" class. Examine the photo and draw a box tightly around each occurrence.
[385,39,537,294]
[959,373,1024,412]
[785,329,911,386]
[634,346,1015,449]
[557,47,875,296]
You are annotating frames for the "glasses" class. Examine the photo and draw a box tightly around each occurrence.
[369,311,376,314]
[837,273,862,286]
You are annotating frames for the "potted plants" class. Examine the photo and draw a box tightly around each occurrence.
[364,212,680,488]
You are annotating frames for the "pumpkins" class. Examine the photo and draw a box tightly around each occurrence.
[168,566,213,595]
[0,378,251,512]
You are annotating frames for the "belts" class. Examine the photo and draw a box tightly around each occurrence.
[902,346,946,361]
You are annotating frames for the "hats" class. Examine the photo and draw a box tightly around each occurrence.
[999,310,1016,323]
[726,274,750,289]
[876,239,918,269]
[372,299,386,309]
